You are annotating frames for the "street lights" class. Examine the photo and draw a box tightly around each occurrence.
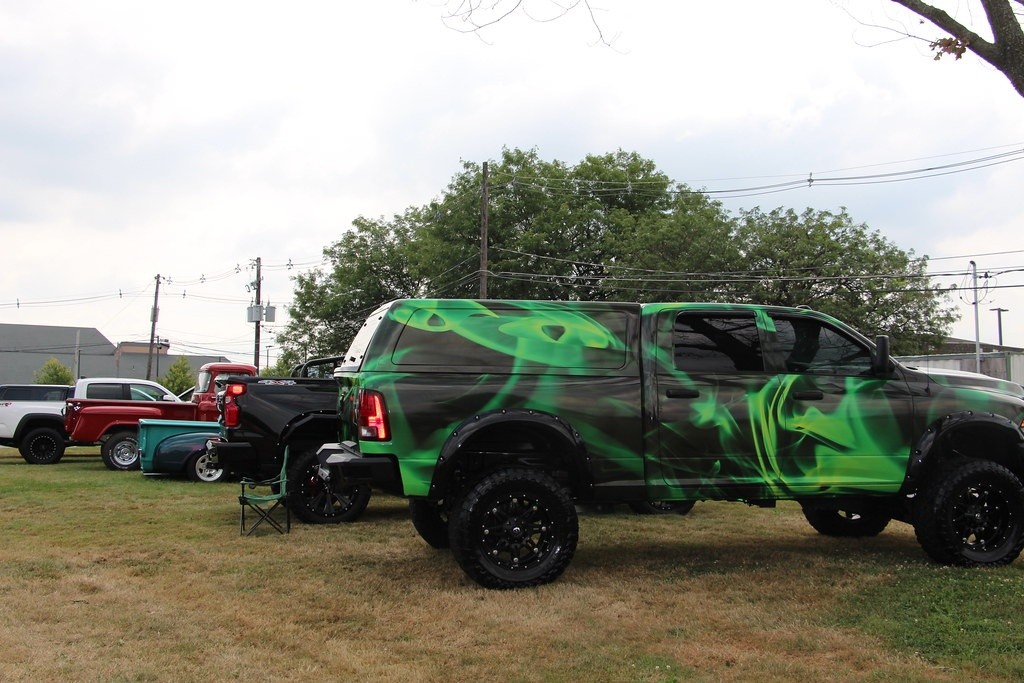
[989,309,1009,345]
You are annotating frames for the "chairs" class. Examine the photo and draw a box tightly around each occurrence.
[240,445,292,536]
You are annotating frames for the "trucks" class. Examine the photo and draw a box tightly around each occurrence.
[137,419,231,482]
[63,363,260,473]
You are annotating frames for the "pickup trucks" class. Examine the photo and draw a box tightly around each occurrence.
[314,299,1023,593]
[0,376,184,466]
[0,384,156,402]
[206,356,372,524]
